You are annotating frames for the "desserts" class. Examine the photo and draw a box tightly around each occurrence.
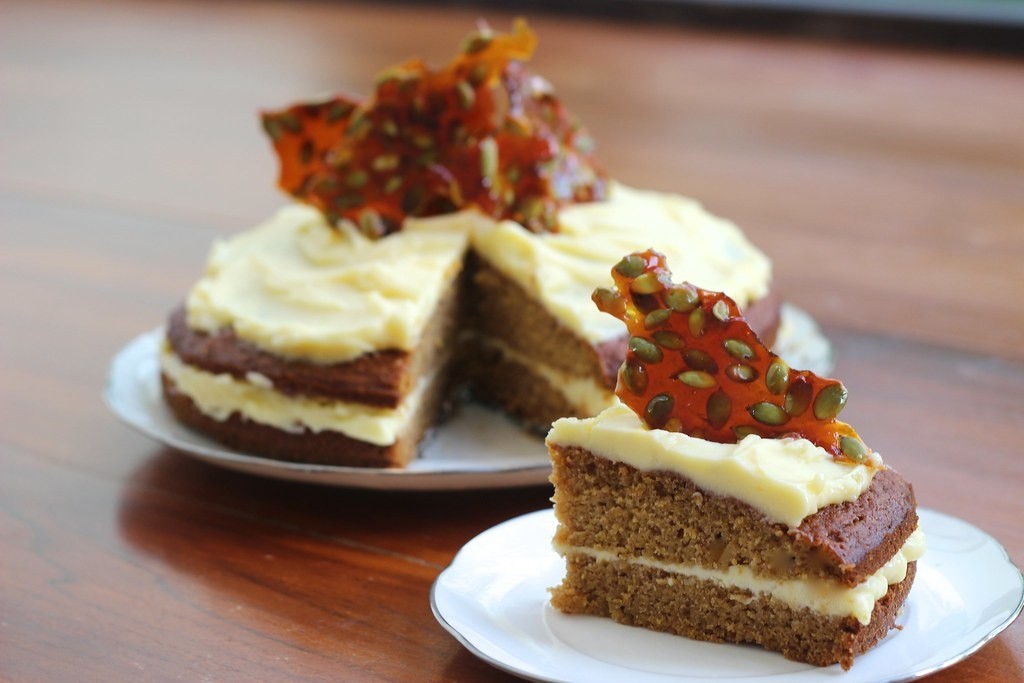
[545,251,923,666]
[160,25,774,468]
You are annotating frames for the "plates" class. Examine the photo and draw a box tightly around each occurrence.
[108,302,835,490]
[429,506,1024,683]
